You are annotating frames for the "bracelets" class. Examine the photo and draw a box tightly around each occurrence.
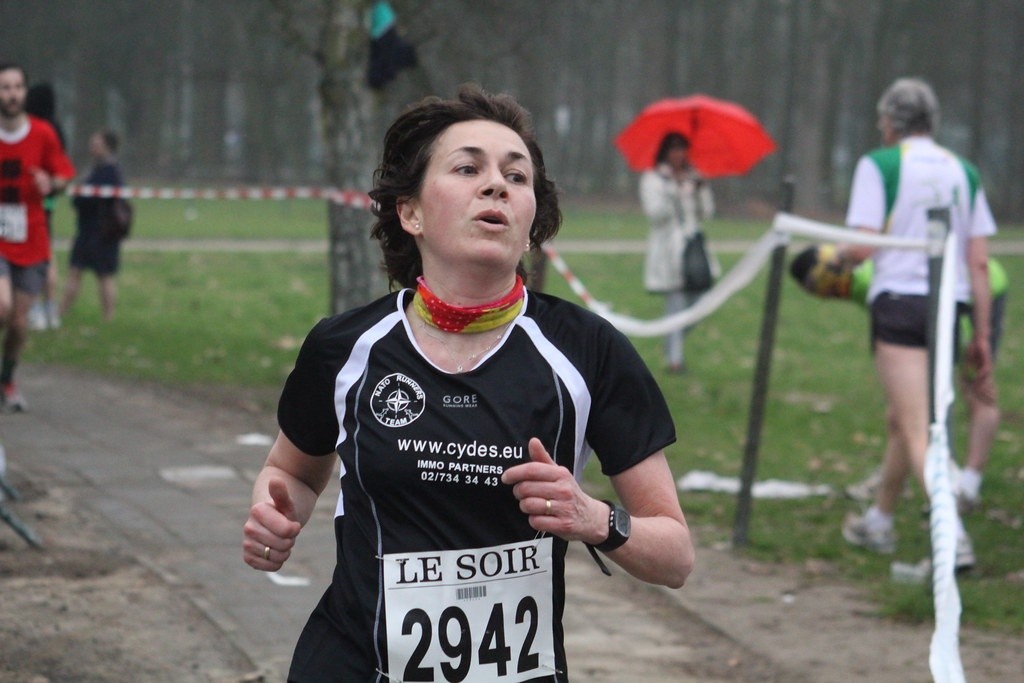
[48,178,57,198]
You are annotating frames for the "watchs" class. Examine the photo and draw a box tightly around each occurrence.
[582,499,632,577]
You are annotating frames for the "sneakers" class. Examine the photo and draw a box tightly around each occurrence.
[953,533,975,569]
[842,512,894,556]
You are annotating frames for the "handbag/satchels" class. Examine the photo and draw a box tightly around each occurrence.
[681,230,710,292]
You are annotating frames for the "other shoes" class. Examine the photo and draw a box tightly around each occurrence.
[846,473,880,501]
[920,494,976,519]
[3,383,26,413]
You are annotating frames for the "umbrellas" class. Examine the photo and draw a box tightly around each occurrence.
[613,94,775,177]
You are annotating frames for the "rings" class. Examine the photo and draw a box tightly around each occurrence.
[263,546,270,560]
[545,499,551,515]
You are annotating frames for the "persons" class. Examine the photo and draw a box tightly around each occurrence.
[829,76,996,581]
[0,64,133,412]
[637,133,716,375]
[242,84,694,683]
[788,243,1011,528]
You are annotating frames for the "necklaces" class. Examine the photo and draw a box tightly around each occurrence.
[419,321,513,372]
